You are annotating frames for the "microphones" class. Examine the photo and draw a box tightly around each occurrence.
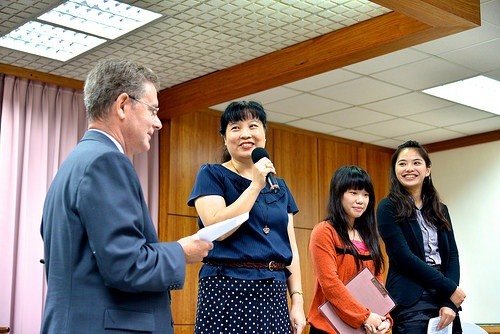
[251,147,280,193]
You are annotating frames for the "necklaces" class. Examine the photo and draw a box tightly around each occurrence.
[231,161,269,234]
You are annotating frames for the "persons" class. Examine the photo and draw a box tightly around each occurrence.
[187,100,307,334]
[40,59,213,334]
[376,140,466,334]
[307,165,394,334]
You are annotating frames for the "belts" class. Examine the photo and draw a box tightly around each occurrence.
[202,257,285,272]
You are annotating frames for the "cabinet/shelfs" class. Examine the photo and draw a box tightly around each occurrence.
[167,111,394,334]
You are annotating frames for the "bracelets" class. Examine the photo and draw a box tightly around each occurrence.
[290,291,303,298]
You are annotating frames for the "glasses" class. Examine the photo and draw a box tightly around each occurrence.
[115,92,159,116]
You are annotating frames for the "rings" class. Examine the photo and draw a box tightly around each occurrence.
[265,164,267,168]
